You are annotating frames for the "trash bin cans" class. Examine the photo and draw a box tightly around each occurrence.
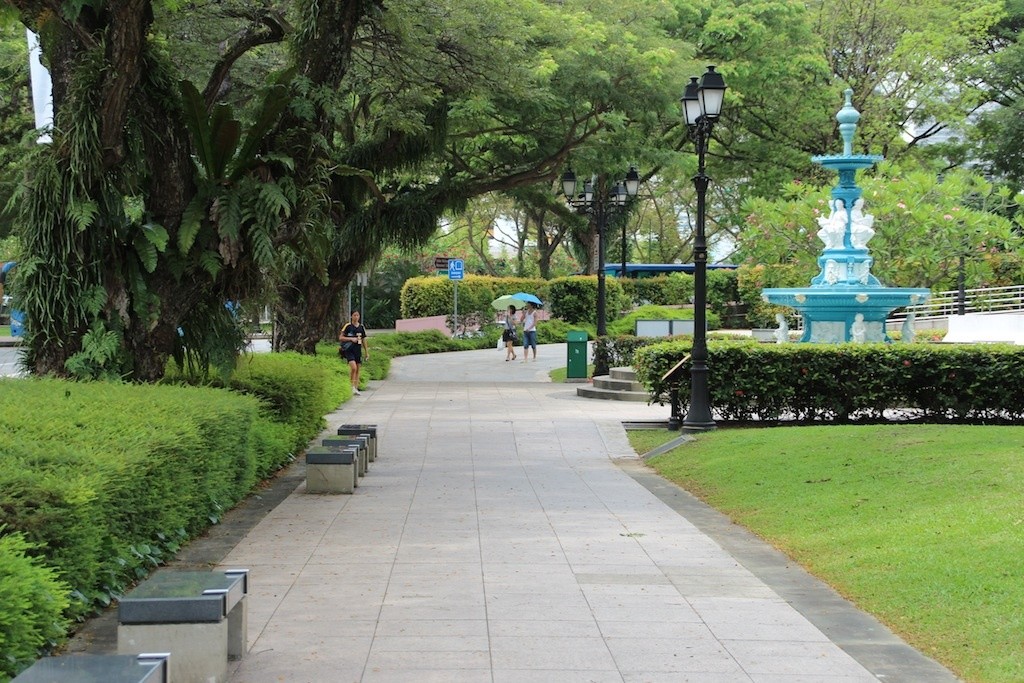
[10,310,28,336]
[567,332,588,377]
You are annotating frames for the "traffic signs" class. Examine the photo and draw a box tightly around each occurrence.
[434,257,456,269]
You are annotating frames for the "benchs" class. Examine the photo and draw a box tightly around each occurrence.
[305,444,361,495]
[7,652,171,683]
[321,433,372,477]
[116,566,250,683]
[336,424,378,462]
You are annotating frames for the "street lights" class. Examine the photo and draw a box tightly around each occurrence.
[957,219,966,315]
[360,271,384,325]
[678,65,729,433]
[559,164,641,377]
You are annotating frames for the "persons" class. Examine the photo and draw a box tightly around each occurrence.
[504,305,518,361]
[340,310,369,395]
[850,199,874,248]
[520,302,537,363]
[817,199,848,250]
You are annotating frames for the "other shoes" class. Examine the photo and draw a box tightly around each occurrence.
[353,388,360,395]
[506,358,509,361]
[512,355,516,360]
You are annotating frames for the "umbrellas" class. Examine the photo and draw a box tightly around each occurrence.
[491,295,524,311]
[511,292,542,311]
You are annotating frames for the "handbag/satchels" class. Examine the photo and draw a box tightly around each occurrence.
[503,329,513,341]
[341,325,354,350]
[497,336,506,351]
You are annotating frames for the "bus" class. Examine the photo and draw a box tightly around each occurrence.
[568,261,752,320]
[0,260,242,338]
